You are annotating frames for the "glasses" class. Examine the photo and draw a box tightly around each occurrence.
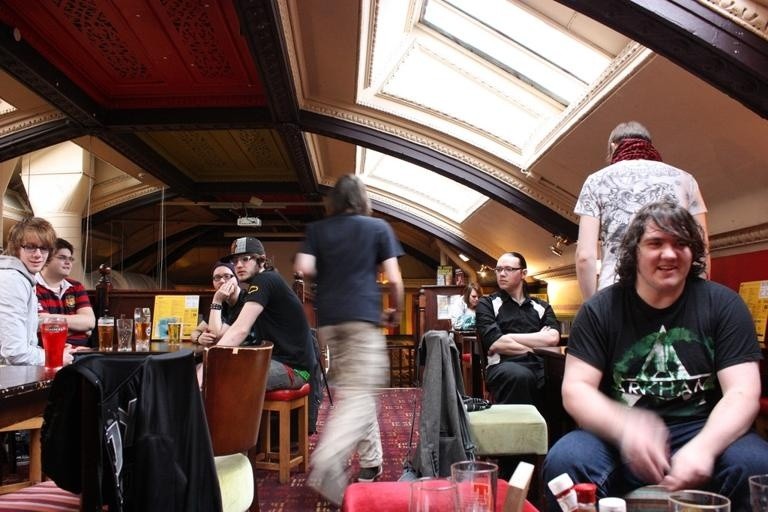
[212,274,234,281]
[20,244,51,253]
[493,266,522,272]
[229,256,253,265]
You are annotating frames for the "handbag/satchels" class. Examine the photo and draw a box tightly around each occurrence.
[397,445,475,481]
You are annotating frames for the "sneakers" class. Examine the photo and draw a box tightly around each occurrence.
[357,466,382,482]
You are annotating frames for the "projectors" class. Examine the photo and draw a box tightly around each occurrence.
[237,216,262,227]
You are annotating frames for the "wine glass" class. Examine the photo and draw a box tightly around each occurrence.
[409,476,462,511]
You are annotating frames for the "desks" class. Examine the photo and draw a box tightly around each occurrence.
[69,340,206,368]
[450,331,485,398]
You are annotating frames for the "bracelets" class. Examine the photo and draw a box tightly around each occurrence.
[209,303,223,310]
[194,332,203,345]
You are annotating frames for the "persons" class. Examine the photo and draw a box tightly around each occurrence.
[540,202,768,512]
[575,121,711,302]
[34,238,95,349]
[195,237,316,392]
[450,281,483,398]
[0,217,74,367]
[191,262,256,347]
[292,174,405,508]
[476,252,575,501]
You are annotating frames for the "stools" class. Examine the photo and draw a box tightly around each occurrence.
[248,383,311,485]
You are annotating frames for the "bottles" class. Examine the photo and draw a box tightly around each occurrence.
[545,471,627,511]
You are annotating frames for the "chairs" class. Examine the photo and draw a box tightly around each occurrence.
[421,330,549,457]
[198,339,274,454]
[340,480,539,512]
[534,347,604,436]
[76,347,254,512]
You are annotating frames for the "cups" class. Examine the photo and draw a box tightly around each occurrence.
[449,460,498,511]
[167,323,182,346]
[667,489,732,511]
[133,307,154,345]
[97,316,115,351]
[748,475,768,511]
[40,318,69,372]
[116,319,134,351]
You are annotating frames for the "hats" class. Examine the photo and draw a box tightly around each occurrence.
[210,259,240,286]
[220,237,264,263]
